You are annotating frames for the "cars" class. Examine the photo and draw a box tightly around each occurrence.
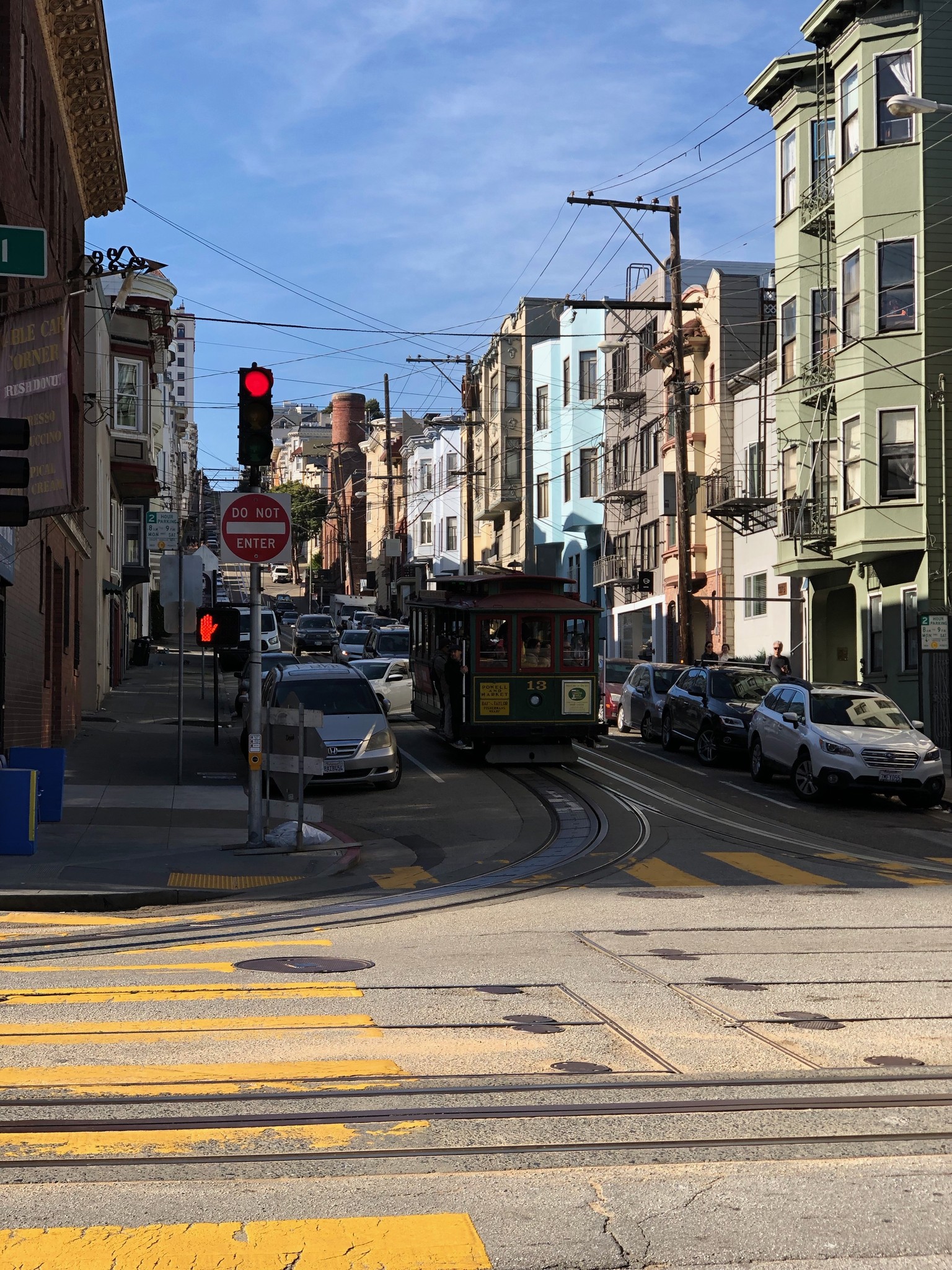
[202,502,220,556]
[203,565,282,653]
[273,594,429,717]
[203,485,212,495]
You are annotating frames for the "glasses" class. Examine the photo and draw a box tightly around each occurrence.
[564,646,572,650]
[707,646,713,649]
[773,647,781,651]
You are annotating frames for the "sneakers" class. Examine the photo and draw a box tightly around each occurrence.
[453,740,466,747]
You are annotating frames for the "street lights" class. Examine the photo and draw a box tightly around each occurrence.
[355,492,398,622]
[597,341,695,666]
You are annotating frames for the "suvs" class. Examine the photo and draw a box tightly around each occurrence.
[269,563,291,583]
[233,654,401,790]
[588,650,946,809]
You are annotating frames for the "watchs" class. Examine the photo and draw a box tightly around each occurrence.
[786,670,789,674]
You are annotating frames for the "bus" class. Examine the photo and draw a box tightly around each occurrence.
[404,573,609,766]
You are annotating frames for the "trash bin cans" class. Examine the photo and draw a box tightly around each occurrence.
[136,636,153,660]
[129,639,148,666]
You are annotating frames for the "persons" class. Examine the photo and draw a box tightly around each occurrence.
[520,638,540,668]
[483,642,507,662]
[444,644,471,748]
[489,619,499,635]
[764,641,792,680]
[400,611,410,626]
[718,644,734,666]
[563,635,589,667]
[701,641,719,667]
[495,620,532,645]
[376,605,390,618]
[537,642,551,668]
[431,635,454,742]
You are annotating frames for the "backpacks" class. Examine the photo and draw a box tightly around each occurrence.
[431,656,447,679]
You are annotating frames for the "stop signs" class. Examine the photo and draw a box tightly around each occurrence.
[220,493,293,563]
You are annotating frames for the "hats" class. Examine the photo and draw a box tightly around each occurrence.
[449,645,462,652]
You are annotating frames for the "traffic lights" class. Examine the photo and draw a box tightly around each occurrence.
[0,417,29,526]
[238,366,274,467]
[196,608,240,646]
